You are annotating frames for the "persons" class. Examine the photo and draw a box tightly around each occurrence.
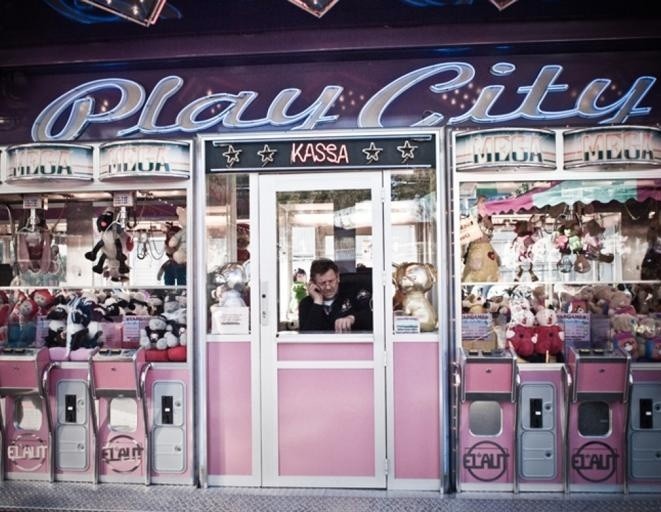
[297,258,375,333]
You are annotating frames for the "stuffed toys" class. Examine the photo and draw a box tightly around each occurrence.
[460,196,661,367]
[392,261,437,331]
[0,194,191,364]
[209,260,251,314]
